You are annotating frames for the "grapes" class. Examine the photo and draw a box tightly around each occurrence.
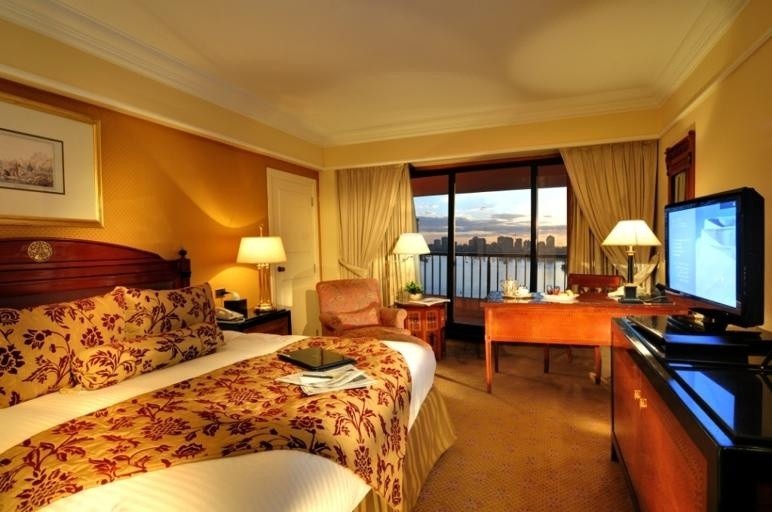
[218,305,292,335]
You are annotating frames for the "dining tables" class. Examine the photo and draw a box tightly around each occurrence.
[406,279,422,299]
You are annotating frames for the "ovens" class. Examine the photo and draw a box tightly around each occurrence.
[0,237,440,511]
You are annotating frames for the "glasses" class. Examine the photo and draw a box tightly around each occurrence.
[236,236,290,313]
[601,219,662,301]
[390,233,430,262]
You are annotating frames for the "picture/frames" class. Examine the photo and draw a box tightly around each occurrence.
[480,290,696,391]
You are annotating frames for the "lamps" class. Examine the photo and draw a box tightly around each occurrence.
[542,274,626,384]
[317,280,411,336]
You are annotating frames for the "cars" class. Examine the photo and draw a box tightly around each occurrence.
[499,279,533,300]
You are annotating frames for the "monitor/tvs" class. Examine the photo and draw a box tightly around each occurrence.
[275,347,378,398]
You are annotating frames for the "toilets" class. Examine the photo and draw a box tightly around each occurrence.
[215,306,244,321]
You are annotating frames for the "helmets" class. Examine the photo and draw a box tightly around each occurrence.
[539,294,581,300]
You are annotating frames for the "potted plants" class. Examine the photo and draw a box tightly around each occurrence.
[1,91,105,230]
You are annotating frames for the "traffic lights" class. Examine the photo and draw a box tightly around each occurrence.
[0,279,226,411]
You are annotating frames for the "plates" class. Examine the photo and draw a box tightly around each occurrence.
[611,313,770,512]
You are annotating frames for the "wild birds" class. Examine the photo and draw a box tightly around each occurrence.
[278,347,356,370]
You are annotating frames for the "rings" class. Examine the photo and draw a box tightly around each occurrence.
[302,374,334,379]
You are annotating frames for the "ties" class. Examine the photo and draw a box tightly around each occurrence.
[664,186,765,335]
